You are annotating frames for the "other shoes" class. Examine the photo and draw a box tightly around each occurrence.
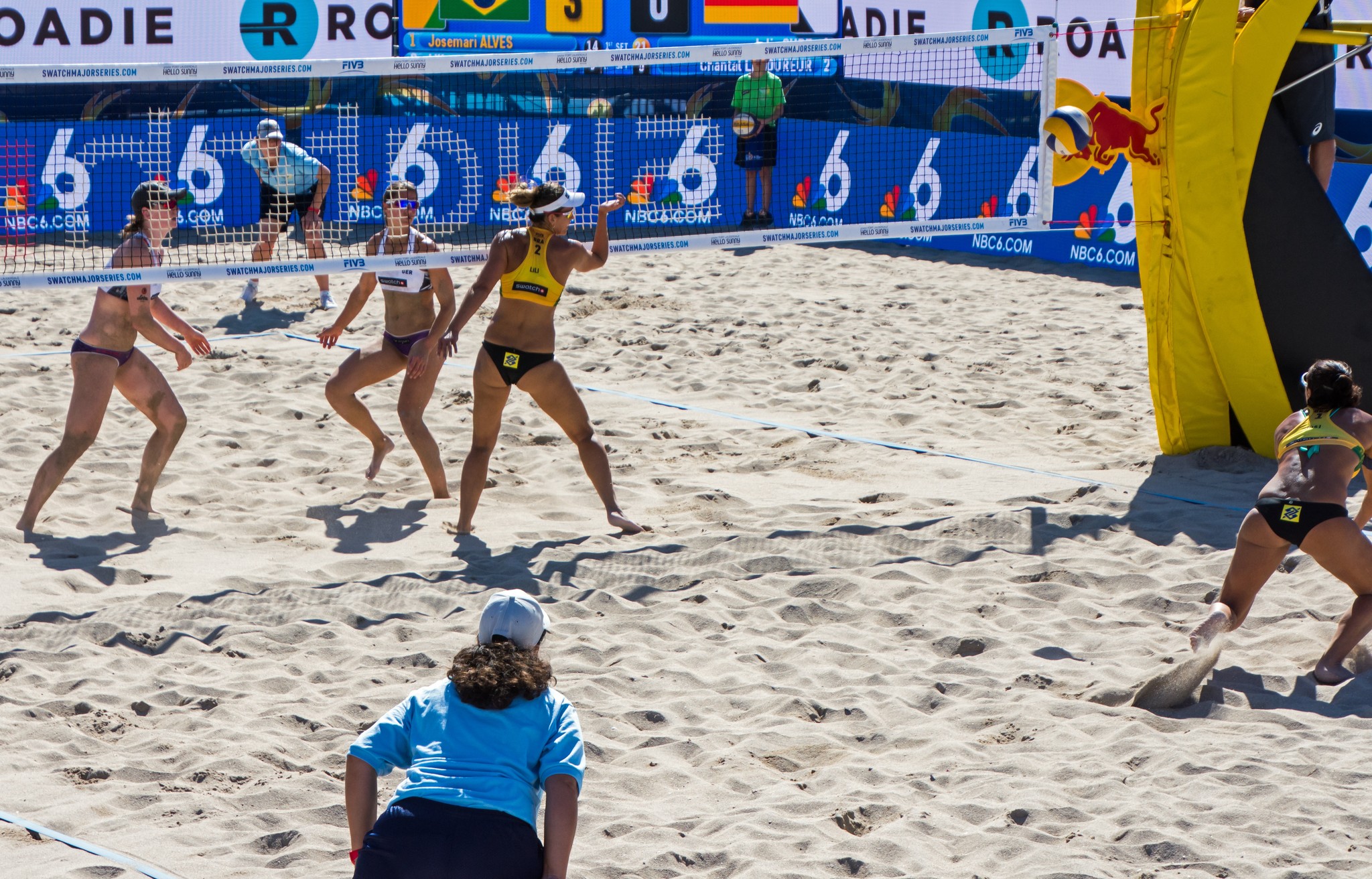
[736,211,755,230]
[755,210,774,230]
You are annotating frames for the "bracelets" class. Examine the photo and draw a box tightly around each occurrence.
[349,849,362,864]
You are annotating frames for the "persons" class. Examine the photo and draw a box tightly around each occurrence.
[732,58,786,221]
[1189,359,1372,684]
[345,588,586,879]
[240,118,335,310]
[438,180,642,532]
[16,179,213,530]
[1237,0,1338,192]
[316,178,456,499]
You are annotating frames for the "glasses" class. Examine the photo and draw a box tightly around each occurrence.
[544,207,576,219]
[383,198,421,209]
[150,199,177,209]
[1302,371,1310,387]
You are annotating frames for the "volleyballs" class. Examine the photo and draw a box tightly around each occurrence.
[732,112,759,139]
[1042,105,1093,156]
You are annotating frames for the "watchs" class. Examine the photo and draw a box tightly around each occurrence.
[307,206,321,214]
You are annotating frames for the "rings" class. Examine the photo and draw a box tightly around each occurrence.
[1243,13,1245,17]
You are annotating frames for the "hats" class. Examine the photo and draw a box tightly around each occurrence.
[478,588,552,652]
[130,180,187,207]
[257,118,283,140]
[526,186,585,215]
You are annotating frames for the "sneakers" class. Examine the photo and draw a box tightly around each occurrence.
[240,284,258,303]
[320,292,338,311]
